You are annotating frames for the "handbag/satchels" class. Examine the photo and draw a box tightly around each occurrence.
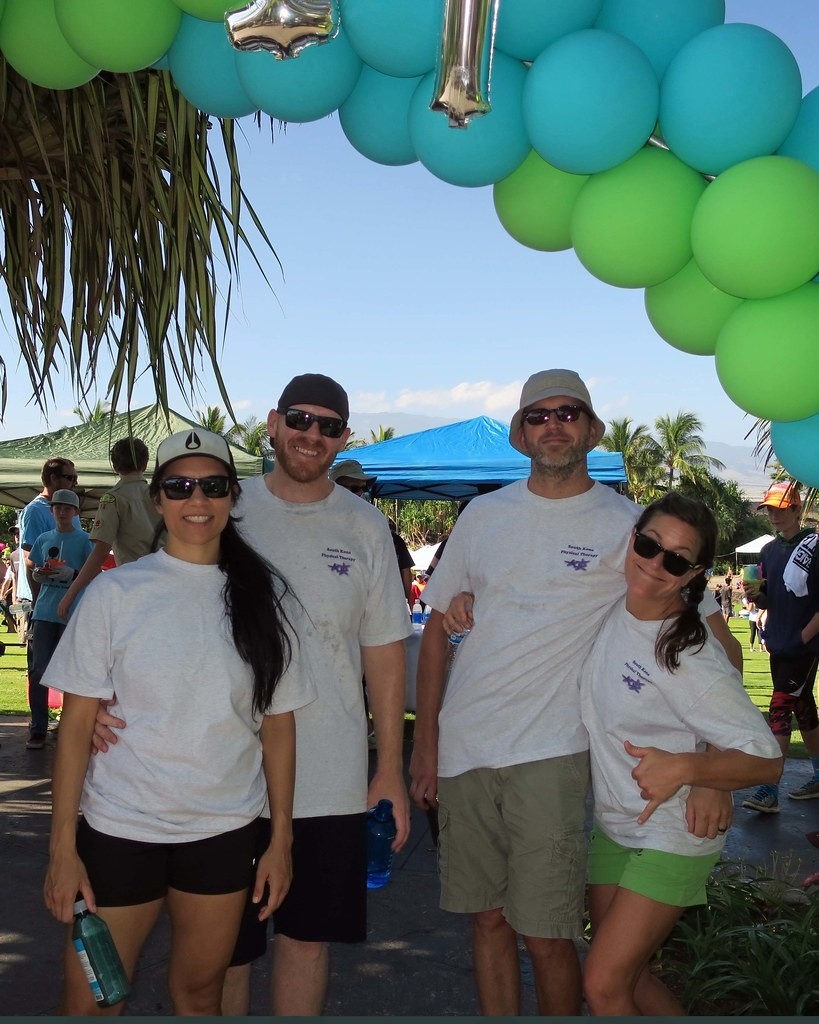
[729,610,735,617]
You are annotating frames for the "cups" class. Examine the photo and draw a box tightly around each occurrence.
[743,564,759,579]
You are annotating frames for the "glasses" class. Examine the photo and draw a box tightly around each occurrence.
[57,474,78,484]
[521,402,587,426]
[278,407,347,438]
[345,484,370,493]
[158,475,234,500]
[13,531,19,536]
[633,527,701,577]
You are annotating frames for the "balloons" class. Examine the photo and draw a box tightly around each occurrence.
[1,1,817,484]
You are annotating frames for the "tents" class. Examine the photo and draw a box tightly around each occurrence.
[0,401,777,572]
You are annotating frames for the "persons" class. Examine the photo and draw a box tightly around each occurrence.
[38,424,319,1015]
[92,374,410,1015]
[410,367,744,1015]
[575,496,785,1017]
[1,439,819,821]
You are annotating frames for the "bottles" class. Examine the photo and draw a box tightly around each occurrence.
[72,898,133,1007]
[447,619,470,668]
[405,599,412,624]
[367,799,396,888]
[412,599,422,630]
[422,603,432,629]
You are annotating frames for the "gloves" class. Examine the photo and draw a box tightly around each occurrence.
[32,567,48,582]
[49,565,74,584]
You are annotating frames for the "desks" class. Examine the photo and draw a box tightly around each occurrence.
[401,619,427,717]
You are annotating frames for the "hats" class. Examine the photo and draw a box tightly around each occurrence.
[329,460,377,484]
[717,583,722,587]
[8,524,19,536]
[509,368,605,458]
[277,374,349,422]
[756,483,802,510]
[153,428,237,480]
[47,489,80,509]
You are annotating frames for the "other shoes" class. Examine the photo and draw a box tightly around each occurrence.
[26,731,45,748]
[750,649,754,652]
[760,649,766,652]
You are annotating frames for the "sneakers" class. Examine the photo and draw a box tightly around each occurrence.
[741,784,780,812]
[788,776,819,799]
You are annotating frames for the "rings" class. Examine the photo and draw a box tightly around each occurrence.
[718,825,728,832]
